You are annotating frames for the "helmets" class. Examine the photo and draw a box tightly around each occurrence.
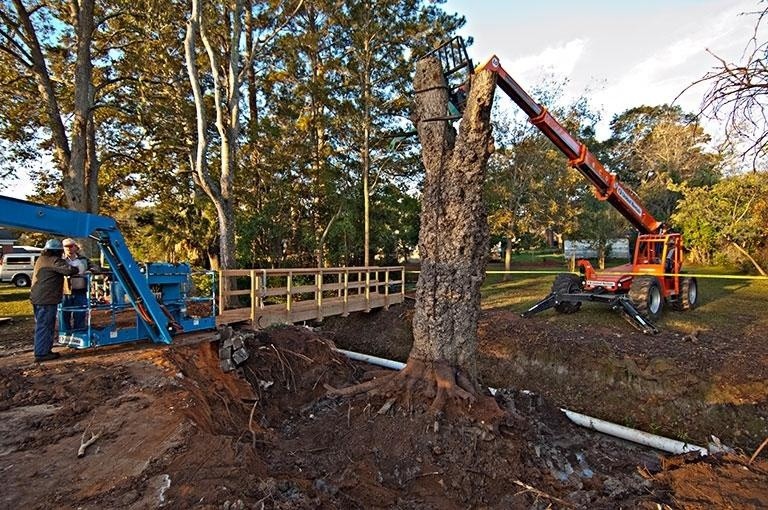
[42,239,64,250]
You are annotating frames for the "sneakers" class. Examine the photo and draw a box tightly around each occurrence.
[35,352,59,362]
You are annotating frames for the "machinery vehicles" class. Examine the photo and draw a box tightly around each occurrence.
[1,193,219,354]
[422,38,699,335]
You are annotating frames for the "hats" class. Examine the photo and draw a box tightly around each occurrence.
[62,239,75,246]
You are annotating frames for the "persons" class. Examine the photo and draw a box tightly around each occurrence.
[59,236,100,335]
[663,240,674,274]
[28,238,64,362]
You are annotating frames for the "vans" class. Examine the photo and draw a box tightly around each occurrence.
[1,252,42,286]
